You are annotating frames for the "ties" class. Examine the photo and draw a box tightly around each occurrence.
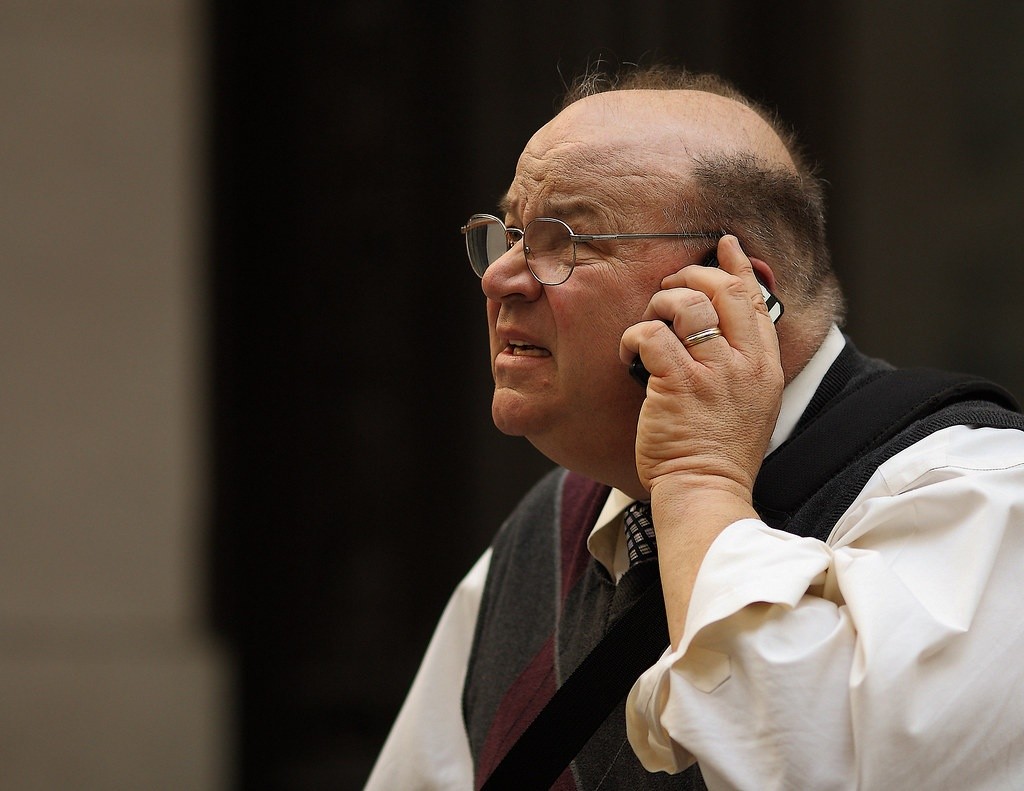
[620,499,656,569]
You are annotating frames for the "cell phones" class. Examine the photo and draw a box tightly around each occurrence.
[629,253,784,386]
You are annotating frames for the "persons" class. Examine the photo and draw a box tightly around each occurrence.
[364,86,1022,789]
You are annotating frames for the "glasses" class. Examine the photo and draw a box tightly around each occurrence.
[461,212,728,286]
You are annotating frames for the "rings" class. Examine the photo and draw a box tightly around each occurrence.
[681,326,724,349]
